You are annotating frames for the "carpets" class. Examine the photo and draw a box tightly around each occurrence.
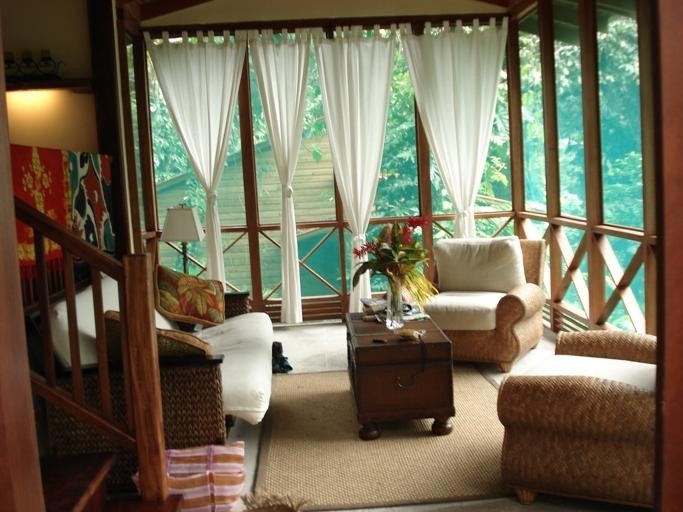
[238,362,514,512]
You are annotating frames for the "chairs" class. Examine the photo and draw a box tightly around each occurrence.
[417,234,546,374]
[495,327,658,508]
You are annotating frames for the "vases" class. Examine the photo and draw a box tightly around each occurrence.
[385,275,405,332]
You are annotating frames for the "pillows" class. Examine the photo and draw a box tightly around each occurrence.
[151,262,226,327]
[433,234,528,296]
[104,309,210,358]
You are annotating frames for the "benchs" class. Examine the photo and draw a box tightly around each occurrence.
[25,270,251,448]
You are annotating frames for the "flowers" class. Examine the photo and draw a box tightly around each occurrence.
[349,214,439,317]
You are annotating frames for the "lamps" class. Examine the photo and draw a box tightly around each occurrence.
[157,203,207,274]
